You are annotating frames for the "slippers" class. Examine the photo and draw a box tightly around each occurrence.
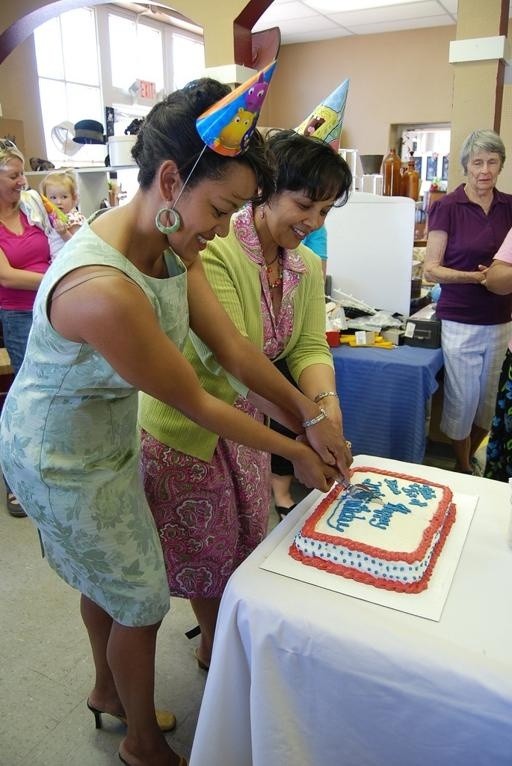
[472,458,483,472]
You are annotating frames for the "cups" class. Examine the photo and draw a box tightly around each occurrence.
[411,259,423,299]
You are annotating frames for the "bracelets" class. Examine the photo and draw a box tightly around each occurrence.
[314,391,338,404]
[300,408,328,428]
[480,269,487,285]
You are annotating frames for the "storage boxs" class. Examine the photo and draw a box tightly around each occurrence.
[405,302,441,349]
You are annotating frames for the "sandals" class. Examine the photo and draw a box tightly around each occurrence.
[275,501,297,523]
[193,647,209,670]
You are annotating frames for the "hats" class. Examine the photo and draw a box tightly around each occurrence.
[72,119,105,144]
[195,58,278,158]
[292,76,351,153]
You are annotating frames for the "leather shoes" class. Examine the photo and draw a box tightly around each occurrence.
[448,462,482,477]
[118,746,187,765]
[86,697,177,731]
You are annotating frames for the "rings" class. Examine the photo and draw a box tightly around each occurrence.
[344,439,352,449]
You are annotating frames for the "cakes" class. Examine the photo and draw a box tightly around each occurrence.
[291,466,455,593]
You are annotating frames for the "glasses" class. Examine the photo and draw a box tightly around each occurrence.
[0,139,17,152]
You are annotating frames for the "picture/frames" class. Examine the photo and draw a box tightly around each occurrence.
[442,156,449,180]
[405,156,421,179]
[426,157,437,181]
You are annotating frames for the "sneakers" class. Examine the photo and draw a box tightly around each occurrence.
[7,492,27,517]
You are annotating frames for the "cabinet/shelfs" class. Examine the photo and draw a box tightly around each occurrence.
[339,148,358,192]
[24,166,140,220]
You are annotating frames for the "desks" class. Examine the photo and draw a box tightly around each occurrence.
[187,454,512,766]
[413,223,427,247]
[423,190,446,213]
[330,343,444,465]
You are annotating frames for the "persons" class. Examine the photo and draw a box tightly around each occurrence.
[0,136,64,515]
[421,128,511,473]
[3,73,355,762]
[39,171,85,263]
[133,127,358,669]
[482,222,512,484]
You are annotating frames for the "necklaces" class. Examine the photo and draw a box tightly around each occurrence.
[465,183,494,211]
[0,208,19,229]
[263,249,283,310]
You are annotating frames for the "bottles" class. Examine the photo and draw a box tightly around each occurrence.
[401,160,419,201]
[382,146,400,196]
